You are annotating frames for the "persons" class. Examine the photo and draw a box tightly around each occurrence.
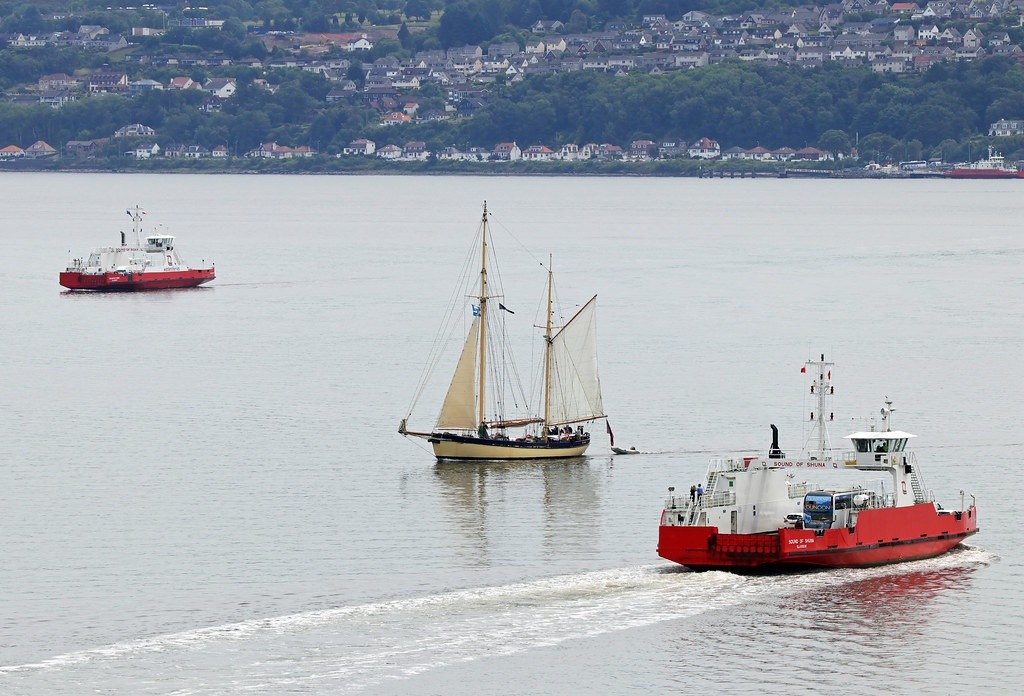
[478,422,490,440]
[882,443,887,451]
[690,483,703,506]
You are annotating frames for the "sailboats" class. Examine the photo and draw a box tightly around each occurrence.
[396,197,609,464]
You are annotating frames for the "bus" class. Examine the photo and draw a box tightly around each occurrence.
[900,160,926,170]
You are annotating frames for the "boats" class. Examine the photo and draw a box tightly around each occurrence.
[59,205,217,293]
[943,150,1024,179]
[656,353,981,577]
[611,446,640,455]
[910,170,946,179]
